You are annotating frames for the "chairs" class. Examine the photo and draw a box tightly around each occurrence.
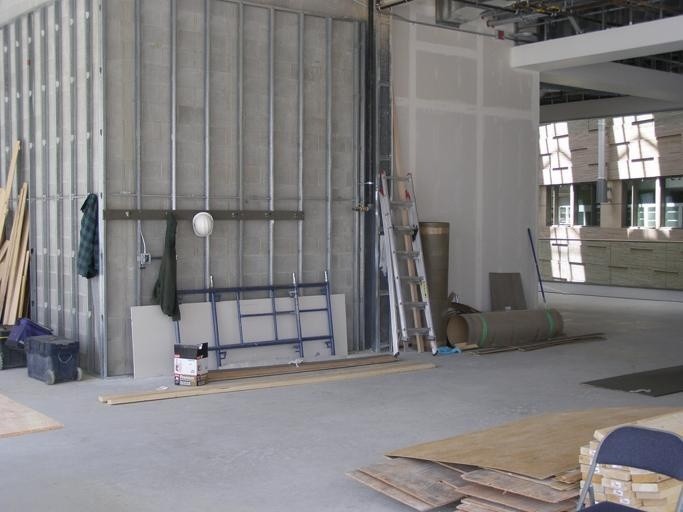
[573,423,682,511]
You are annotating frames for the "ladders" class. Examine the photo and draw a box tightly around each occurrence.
[376,170,438,359]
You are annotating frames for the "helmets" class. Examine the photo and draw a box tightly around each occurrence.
[192,212,214,237]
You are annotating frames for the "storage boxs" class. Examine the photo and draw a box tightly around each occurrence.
[0,317,83,387]
